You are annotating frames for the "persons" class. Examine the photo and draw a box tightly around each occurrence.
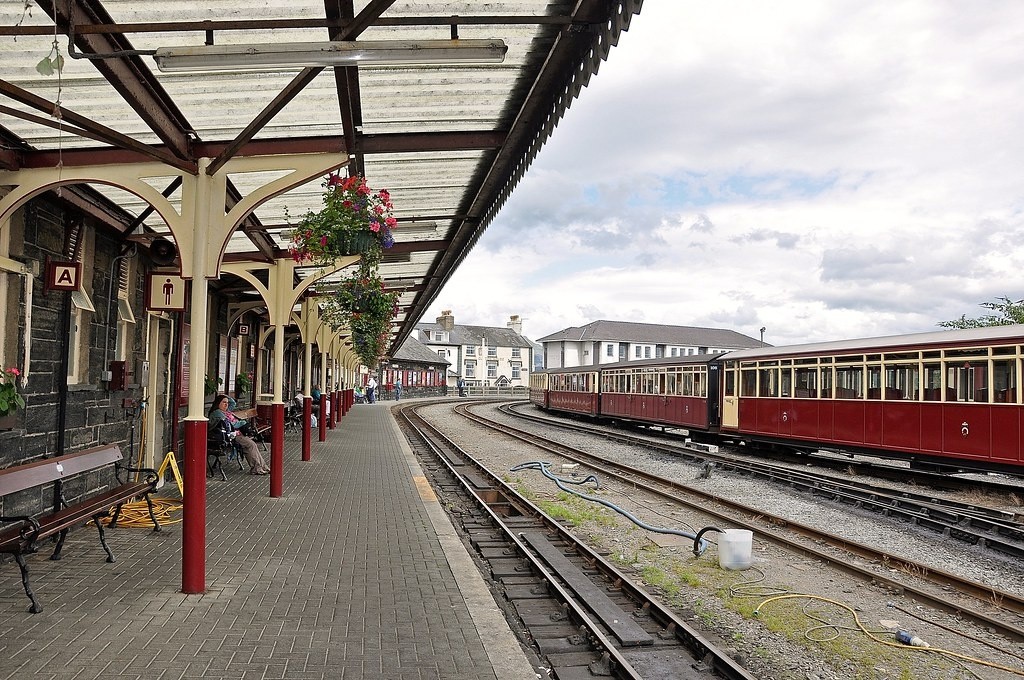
[295,375,382,427]
[207,394,270,475]
[396,377,402,401]
[458,378,466,397]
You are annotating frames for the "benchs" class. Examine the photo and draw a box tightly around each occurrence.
[794,386,1017,403]
[284,406,303,436]
[0,443,162,616]
[206,408,272,482]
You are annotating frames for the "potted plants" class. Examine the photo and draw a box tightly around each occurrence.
[204,370,223,404]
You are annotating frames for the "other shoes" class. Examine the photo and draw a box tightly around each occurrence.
[250,469,268,474]
[263,465,270,474]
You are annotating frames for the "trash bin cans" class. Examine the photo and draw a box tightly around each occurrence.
[257,393,274,438]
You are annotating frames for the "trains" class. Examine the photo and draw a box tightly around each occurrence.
[530,323,1023,478]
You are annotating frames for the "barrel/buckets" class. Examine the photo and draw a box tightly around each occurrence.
[718,529,753,571]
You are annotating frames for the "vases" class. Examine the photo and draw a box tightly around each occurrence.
[343,298,369,313]
[333,229,373,257]
[354,323,372,334]
[0,416,17,431]
[237,393,249,403]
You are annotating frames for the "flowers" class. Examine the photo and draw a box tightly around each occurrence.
[283,171,408,370]
[236,371,253,394]
[0,365,25,417]
